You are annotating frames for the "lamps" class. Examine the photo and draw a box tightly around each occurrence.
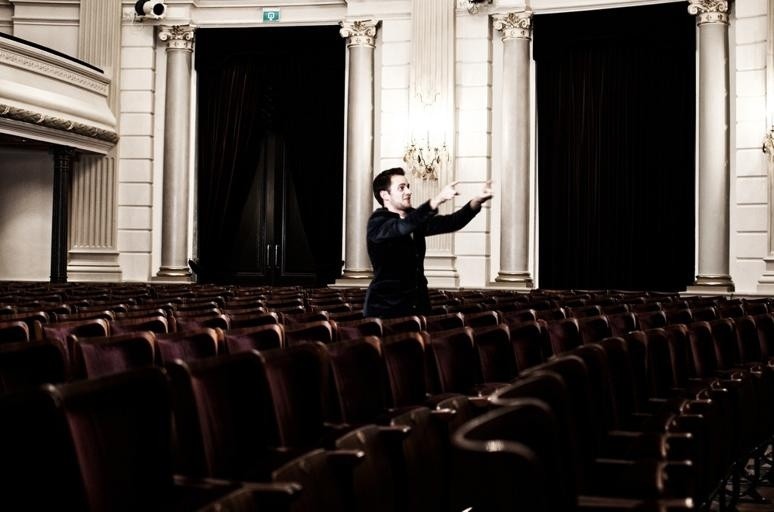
[403,140,448,182]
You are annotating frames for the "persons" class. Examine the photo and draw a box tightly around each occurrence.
[364,169,492,314]
